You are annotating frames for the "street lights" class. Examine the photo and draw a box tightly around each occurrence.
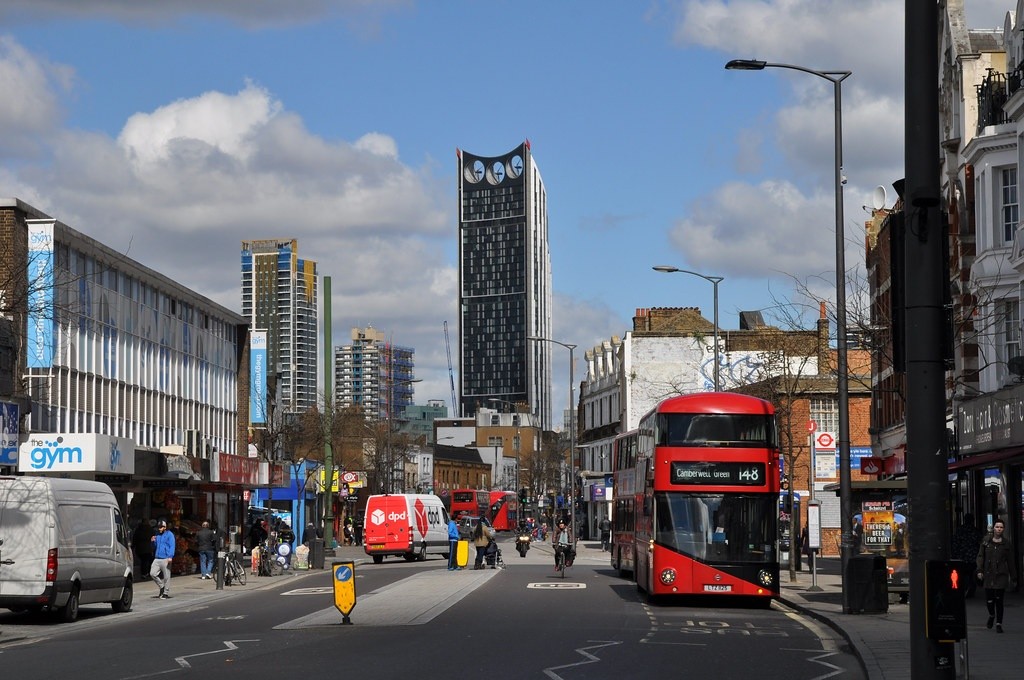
[489,398,520,526]
[527,335,575,551]
[724,57,853,614]
[653,265,724,392]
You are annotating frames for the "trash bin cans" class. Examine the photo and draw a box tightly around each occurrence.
[308,537,325,569]
[845,554,889,616]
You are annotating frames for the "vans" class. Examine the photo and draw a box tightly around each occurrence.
[0,475,134,624]
[455,515,496,542]
[362,494,460,565]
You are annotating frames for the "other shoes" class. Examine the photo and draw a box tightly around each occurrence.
[158,587,164,597]
[202,576,208,580]
[474,564,484,569]
[160,594,168,599]
[566,561,570,567]
[448,567,461,571]
[555,566,559,572]
[206,573,212,579]
[987,616,995,629]
[997,625,1003,633]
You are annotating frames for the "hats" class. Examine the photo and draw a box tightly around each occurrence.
[202,522,210,527]
[158,521,166,527]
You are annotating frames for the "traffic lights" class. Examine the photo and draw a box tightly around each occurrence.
[925,557,968,645]
[519,488,527,503]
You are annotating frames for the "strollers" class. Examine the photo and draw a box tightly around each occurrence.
[483,538,507,570]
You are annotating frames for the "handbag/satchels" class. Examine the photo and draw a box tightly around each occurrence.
[976,576,983,587]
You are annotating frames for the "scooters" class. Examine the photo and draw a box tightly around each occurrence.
[513,528,534,558]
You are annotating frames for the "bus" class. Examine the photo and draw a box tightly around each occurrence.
[611,427,638,579]
[633,391,789,605]
[449,488,517,533]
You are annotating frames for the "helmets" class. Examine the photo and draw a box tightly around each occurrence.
[559,519,565,524]
[519,519,526,526]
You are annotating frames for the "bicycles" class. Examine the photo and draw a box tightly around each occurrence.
[213,548,247,586]
[256,540,285,577]
[554,541,570,579]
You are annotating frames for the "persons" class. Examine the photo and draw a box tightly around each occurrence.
[302,521,322,544]
[977,519,1018,636]
[448,514,461,572]
[150,520,175,600]
[247,516,295,566]
[801,521,819,573]
[953,515,981,599]
[575,517,582,541]
[884,523,904,559]
[196,522,218,580]
[552,519,576,572]
[526,517,548,542]
[481,514,491,527]
[516,519,532,552]
[344,521,363,547]
[599,515,611,552]
[472,518,492,570]
[852,518,865,556]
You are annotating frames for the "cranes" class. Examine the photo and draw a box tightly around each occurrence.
[443,320,459,418]
